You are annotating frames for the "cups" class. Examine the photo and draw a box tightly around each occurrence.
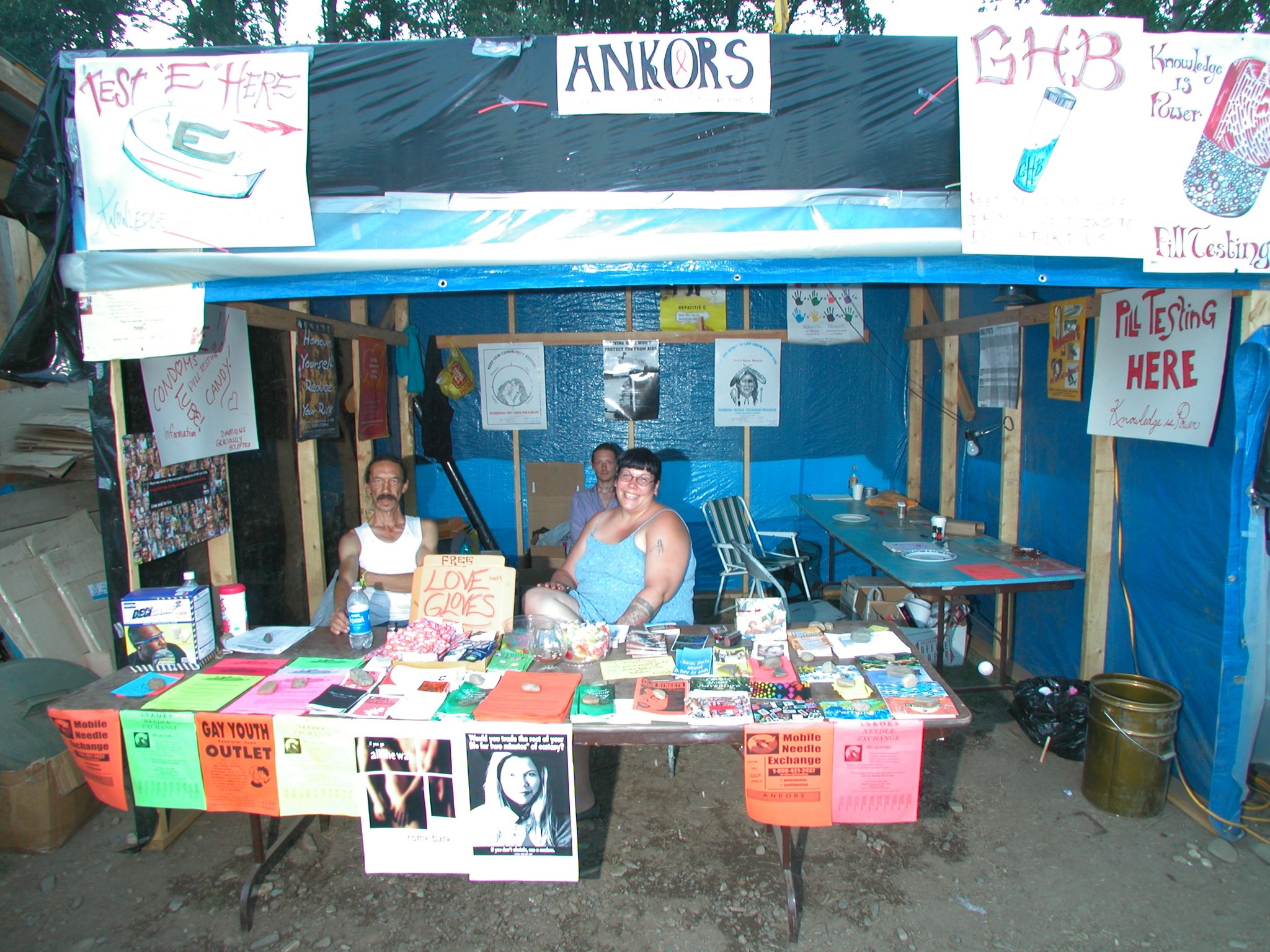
[218,583,248,638]
[851,483,864,500]
[931,515,947,543]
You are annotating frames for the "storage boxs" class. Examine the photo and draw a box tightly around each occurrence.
[434,517,462,532]
[121,585,218,673]
[515,533,567,600]
[0,749,106,853]
[840,576,968,666]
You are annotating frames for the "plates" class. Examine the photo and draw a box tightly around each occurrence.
[832,514,870,522]
[901,549,958,563]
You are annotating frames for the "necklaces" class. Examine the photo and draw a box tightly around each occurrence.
[596,487,613,509]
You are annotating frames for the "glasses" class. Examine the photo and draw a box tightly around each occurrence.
[616,469,656,487]
[134,632,165,645]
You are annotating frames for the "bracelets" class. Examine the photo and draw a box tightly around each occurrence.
[359,570,367,588]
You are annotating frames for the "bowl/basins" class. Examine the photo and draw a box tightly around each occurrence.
[504,615,556,654]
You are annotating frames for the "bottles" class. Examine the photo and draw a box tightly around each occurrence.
[346,582,373,650]
[183,570,201,588]
[897,501,906,519]
[848,465,858,495]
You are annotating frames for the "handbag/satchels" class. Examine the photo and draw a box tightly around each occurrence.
[434,334,476,399]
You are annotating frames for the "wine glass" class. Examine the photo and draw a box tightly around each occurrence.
[529,623,567,673]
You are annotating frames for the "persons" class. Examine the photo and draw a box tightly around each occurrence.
[329,454,440,636]
[471,749,571,852]
[570,442,620,556]
[122,432,230,565]
[356,741,455,829]
[521,447,697,626]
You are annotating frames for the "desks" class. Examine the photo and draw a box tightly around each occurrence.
[792,495,1086,692]
[47,620,972,943]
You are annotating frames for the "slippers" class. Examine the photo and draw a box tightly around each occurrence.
[575,804,600,820]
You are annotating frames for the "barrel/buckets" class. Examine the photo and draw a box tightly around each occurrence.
[1080,673,1183,819]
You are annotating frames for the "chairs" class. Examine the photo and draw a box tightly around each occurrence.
[703,496,850,623]
